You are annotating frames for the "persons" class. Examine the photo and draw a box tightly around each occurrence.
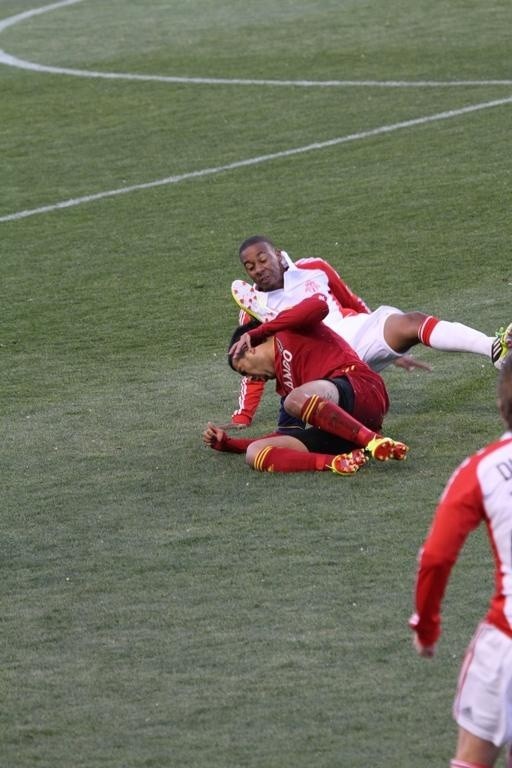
[201,296,411,477]
[409,353,511,767]
[205,234,512,433]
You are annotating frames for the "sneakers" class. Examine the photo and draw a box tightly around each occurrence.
[491,323,512,371]
[365,438,410,461]
[331,447,370,477]
[231,279,279,323]
